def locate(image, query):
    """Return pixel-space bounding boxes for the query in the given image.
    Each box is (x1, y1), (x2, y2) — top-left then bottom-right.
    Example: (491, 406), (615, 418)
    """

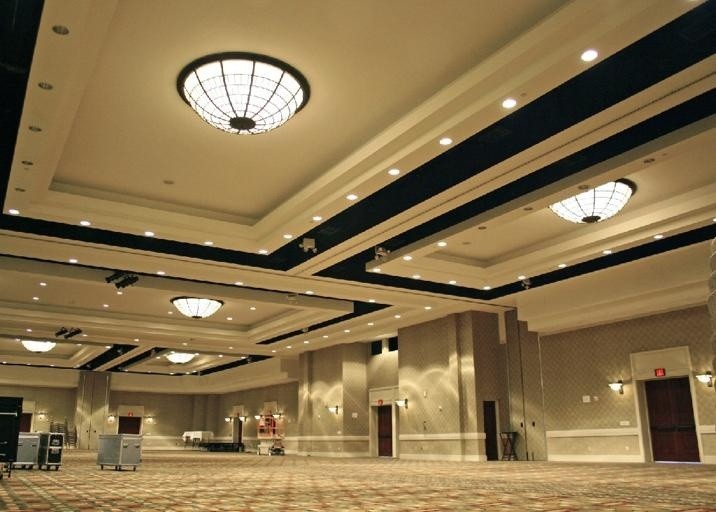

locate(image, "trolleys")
(1, 412), (64, 478)
(96, 432), (143, 471)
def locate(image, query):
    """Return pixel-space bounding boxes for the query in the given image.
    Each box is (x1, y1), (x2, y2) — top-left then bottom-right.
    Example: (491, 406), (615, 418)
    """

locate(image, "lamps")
(607, 379), (625, 395)
(175, 50), (311, 136)
(545, 177), (639, 225)
(696, 371), (713, 387)
(169, 296), (225, 320)
